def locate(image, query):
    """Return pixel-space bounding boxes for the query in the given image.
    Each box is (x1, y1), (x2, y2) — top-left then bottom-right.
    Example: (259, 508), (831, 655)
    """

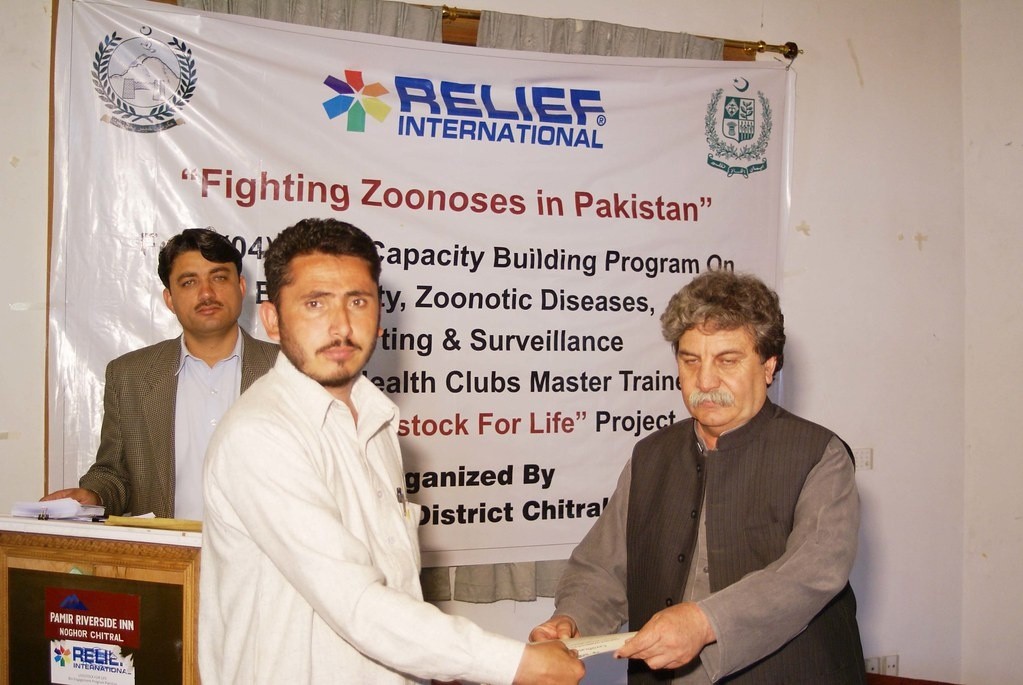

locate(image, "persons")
(39, 229), (281, 522)
(196, 218), (586, 685)
(528, 272), (866, 685)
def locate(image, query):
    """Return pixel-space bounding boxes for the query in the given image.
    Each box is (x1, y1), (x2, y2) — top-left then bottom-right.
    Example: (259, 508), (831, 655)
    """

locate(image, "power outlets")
(864, 654), (898, 677)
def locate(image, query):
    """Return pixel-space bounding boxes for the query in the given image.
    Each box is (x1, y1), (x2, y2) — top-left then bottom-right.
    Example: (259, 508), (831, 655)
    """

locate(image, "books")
(533, 631), (638, 659)
